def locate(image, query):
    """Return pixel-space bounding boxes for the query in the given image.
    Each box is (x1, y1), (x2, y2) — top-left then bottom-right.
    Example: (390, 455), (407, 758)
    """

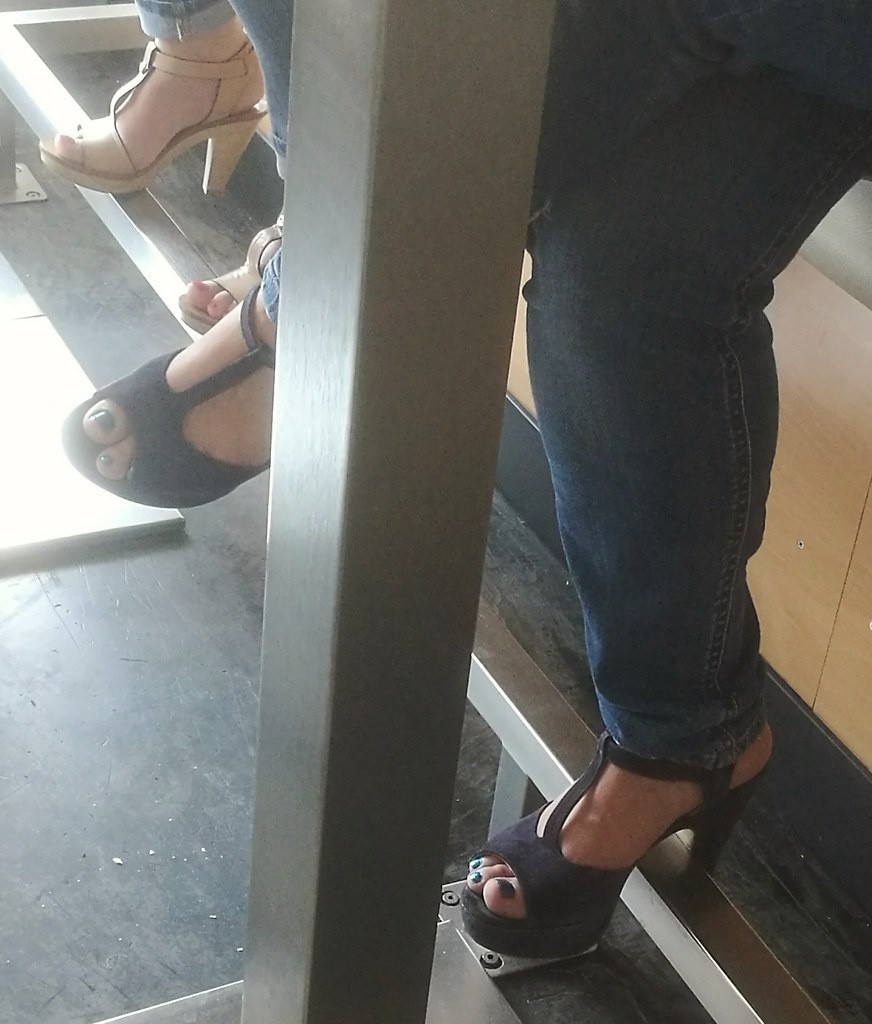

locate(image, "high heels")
(461, 716), (774, 957)
(63, 287), (275, 510)
(178, 216), (284, 336)
(39, 35), (271, 198)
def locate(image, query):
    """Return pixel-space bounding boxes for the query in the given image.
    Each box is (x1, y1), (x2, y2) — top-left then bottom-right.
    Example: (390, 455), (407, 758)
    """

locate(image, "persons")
(61, 0), (872, 961)
(38, 0), (293, 337)
